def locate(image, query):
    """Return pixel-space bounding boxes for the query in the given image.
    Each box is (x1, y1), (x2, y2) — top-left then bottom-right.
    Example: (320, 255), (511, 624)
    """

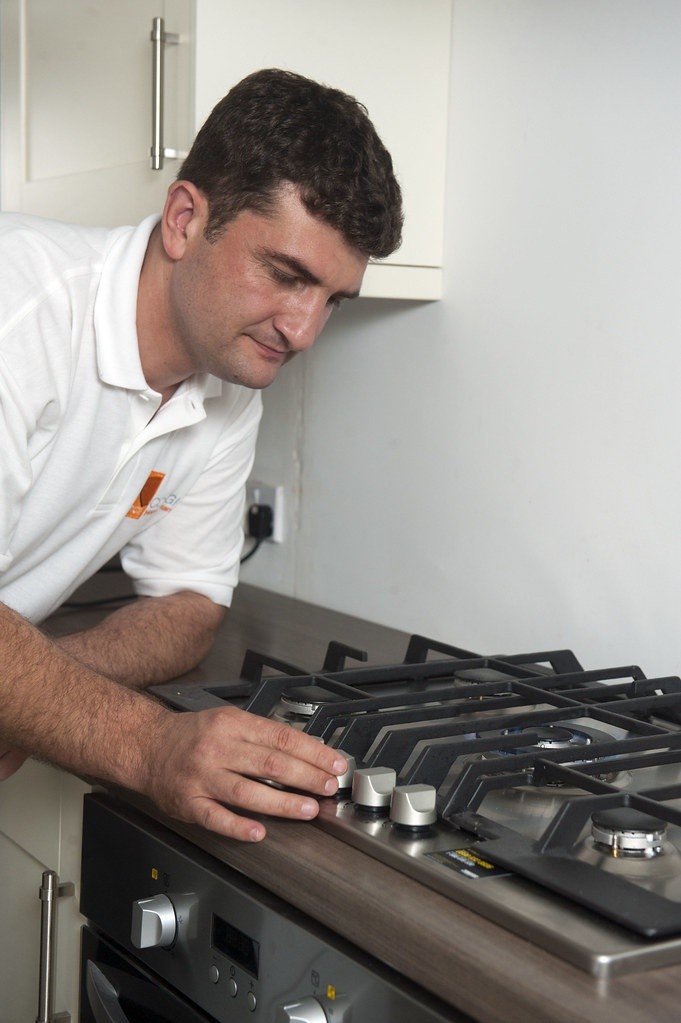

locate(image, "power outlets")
(239, 477), (287, 545)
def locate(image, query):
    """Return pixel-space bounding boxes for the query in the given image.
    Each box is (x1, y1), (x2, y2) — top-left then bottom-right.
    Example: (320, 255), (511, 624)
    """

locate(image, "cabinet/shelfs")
(1, 559), (451, 1023)
(0, 1), (446, 303)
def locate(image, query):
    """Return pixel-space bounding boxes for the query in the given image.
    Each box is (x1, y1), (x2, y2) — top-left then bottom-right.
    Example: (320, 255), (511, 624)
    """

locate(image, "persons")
(0, 67), (405, 844)
